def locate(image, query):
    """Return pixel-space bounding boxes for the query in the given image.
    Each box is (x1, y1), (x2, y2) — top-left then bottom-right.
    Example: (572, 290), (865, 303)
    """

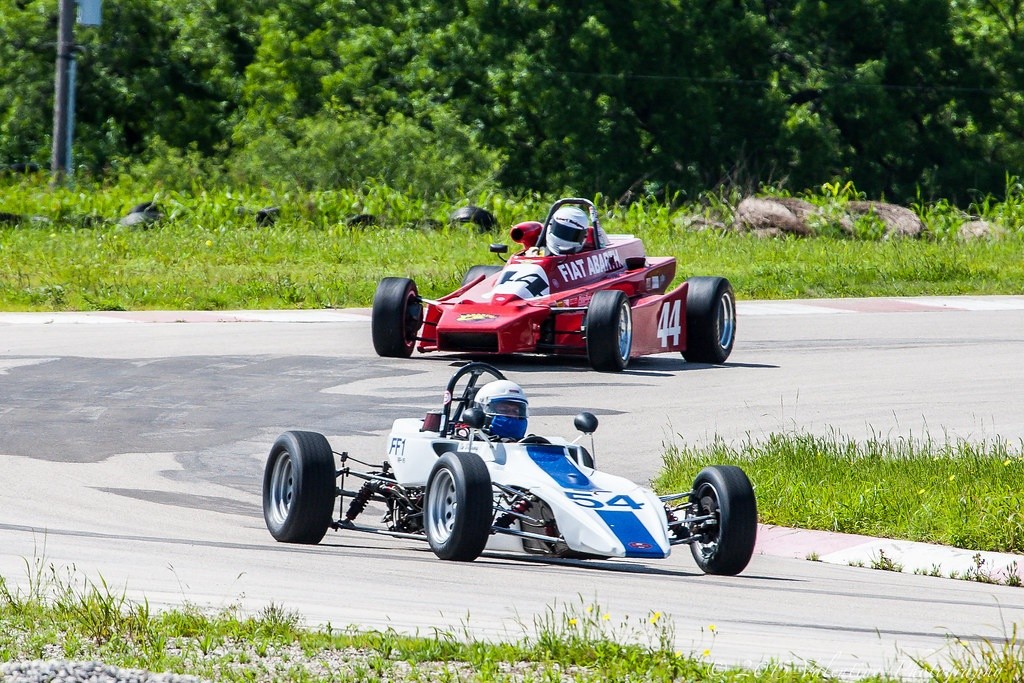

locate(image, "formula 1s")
(262, 363), (758, 577)
(372, 197), (736, 374)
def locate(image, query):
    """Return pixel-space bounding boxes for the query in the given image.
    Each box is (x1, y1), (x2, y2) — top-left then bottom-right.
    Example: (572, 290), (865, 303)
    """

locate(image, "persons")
(525, 207), (589, 257)
(454, 380), (528, 441)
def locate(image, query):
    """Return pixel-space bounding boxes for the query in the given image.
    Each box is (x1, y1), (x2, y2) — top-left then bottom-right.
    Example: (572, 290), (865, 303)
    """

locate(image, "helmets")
(546, 206), (589, 256)
(473, 380), (529, 440)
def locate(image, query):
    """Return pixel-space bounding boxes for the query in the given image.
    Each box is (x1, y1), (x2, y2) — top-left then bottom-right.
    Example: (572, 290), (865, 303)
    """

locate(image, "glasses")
(494, 408), (520, 414)
